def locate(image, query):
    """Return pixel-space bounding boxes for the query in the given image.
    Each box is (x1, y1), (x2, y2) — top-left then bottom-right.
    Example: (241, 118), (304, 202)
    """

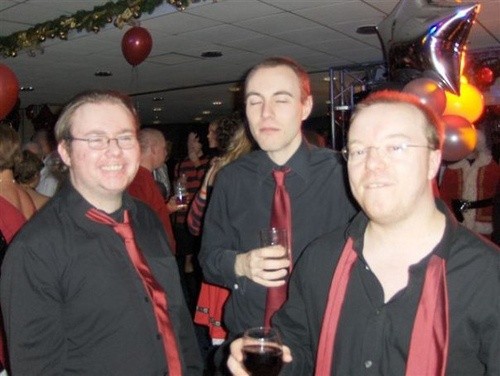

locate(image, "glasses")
(67, 134), (136, 150)
(341, 142), (432, 162)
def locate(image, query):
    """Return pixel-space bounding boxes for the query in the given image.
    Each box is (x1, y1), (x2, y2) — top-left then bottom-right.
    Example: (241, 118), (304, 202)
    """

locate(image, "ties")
(264, 168), (293, 330)
(86, 208), (181, 376)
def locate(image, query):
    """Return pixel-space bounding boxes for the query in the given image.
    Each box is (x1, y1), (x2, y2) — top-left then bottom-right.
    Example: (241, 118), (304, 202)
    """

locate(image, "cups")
(242, 327), (284, 376)
(260, 226), (288, 271)
(176, 193), (189, 210)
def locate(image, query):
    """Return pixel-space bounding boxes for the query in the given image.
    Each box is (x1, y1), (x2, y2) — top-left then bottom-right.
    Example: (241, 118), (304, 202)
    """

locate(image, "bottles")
(176, 174), (187, 193)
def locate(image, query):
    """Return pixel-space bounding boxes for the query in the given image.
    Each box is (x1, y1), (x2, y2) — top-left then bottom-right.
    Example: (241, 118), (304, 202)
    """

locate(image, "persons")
(0, 89), (203, 376)
(0, 110), (259, 376)
(213, 91), (499, 376)
(198, 57), (362, 341)
(440, 125), (499, 241)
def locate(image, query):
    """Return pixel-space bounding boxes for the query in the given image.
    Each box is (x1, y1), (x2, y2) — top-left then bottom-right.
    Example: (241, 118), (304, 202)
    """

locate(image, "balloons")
(375, 1), (497, 162)
